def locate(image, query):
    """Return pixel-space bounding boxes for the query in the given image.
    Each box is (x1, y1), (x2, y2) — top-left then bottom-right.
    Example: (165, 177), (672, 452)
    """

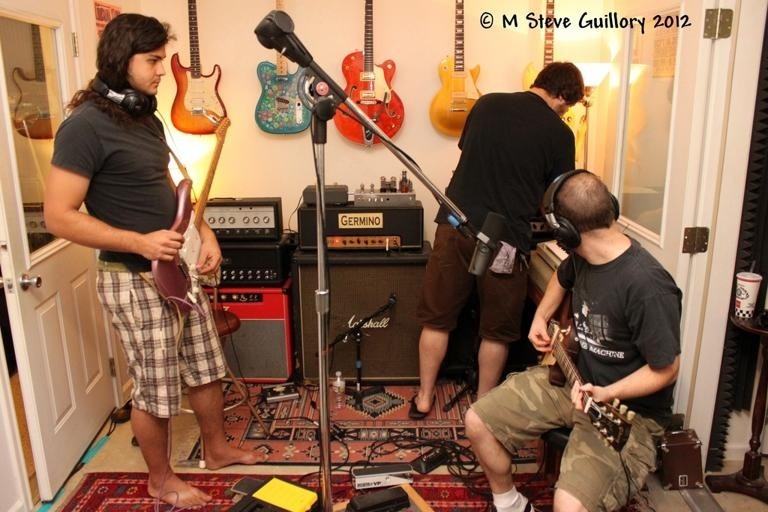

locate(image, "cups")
(736, 273), (763, 320)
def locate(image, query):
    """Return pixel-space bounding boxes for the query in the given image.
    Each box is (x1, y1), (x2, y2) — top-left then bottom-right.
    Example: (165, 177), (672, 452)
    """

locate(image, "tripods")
(315, 304), (385, 411)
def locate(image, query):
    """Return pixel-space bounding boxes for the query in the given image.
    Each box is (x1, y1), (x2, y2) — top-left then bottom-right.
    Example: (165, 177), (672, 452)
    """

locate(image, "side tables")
(705, 301), (768, 504)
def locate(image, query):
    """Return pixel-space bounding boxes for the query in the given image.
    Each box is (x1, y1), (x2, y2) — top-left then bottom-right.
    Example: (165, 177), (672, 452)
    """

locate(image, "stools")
(540, 427), (575, 479)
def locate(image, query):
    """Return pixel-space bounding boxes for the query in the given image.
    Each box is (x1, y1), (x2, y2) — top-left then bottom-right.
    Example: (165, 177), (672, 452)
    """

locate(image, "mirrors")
(1, 8), (78, 271)
(610, 4), (685, 250)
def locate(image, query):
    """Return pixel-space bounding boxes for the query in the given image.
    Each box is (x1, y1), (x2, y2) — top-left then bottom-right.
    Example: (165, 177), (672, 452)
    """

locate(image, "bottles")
(333, 371), (347, 410)
(380, 170), (410, 193)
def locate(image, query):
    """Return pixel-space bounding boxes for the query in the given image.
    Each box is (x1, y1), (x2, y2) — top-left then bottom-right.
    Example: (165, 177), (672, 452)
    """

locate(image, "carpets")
(173, 381), (545, 464)
(47, 471), (656, 512)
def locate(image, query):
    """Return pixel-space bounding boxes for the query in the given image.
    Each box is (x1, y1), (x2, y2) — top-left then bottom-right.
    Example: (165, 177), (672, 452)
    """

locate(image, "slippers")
(408, 393), (436, 420)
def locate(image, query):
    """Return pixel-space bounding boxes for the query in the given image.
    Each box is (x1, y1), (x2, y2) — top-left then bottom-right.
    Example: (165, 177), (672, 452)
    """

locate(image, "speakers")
(293, 240), (433, 386)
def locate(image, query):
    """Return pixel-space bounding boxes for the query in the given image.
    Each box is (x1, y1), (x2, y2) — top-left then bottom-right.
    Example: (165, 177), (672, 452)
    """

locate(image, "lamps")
(629, 63), (647, 84)
(575, 61), (611, 170)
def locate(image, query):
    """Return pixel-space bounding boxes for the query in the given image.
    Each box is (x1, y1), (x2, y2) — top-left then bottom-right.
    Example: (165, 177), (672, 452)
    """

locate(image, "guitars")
(254, 1), (314, 134)
(521, 0), (577, 127)
(430, 0), (483, 138)
(335, 0), (404, 146)
(169, 1), (227, 136)
(536, 316), (636, 452)
(12, 23), (54, 140)
(148, 117), (232, 311)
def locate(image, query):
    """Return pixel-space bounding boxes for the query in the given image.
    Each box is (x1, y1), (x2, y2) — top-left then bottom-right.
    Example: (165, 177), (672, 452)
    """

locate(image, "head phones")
(91, 70), (157, 118)
(543, 168), (619, 250)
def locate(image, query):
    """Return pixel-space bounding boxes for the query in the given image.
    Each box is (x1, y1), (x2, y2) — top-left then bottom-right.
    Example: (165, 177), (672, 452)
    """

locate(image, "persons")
(464, 167), (685, 512)
(41, 13), (265, 511)
(405, 60), (588, 425)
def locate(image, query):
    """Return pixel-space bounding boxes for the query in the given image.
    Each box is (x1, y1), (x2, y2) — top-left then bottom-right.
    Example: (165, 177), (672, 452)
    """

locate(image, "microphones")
(467, 211), (509, 278)
(388, 296), (396, 310)
